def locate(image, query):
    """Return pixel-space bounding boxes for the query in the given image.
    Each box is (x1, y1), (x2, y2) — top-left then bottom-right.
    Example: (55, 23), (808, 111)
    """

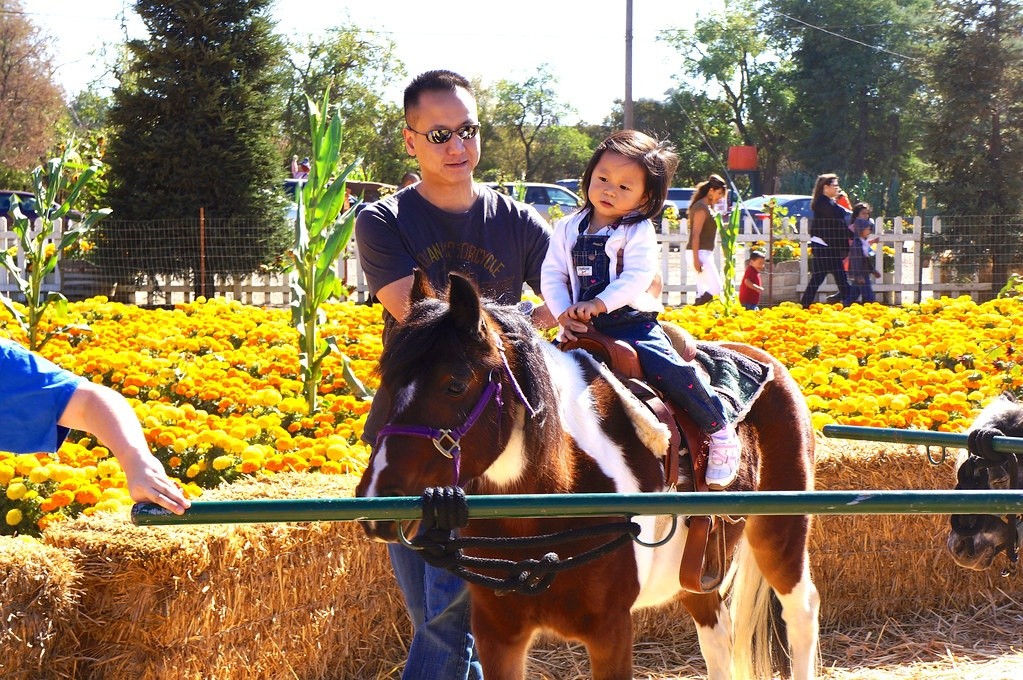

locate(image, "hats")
(299, 157), (310, 169)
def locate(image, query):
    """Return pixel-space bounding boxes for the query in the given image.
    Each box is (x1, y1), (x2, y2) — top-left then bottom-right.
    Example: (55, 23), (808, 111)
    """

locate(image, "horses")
(948, 395), (1023, 573)
(356, 267), (820, 680)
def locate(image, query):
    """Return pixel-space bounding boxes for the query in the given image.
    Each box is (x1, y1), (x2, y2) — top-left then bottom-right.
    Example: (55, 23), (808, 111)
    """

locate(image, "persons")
(540, 130), (743, 490)
(799, 172), (857, 310)
(738, 251), (767, 311)
(847, 217), (880, 307)
(355, 69), (555, 680)
(291, 155), (311, 180)
(824, 204), (880, 302)
(0, 336), (191, 515)
(401, 172), (419, 188)
(686, 174), (727, 301)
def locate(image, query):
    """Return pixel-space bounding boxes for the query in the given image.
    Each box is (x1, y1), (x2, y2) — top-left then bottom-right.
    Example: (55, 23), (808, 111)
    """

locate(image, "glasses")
(406, 124), (482, 144)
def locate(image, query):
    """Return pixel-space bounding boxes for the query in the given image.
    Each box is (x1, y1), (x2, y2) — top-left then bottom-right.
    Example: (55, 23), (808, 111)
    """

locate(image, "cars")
(730, 190), (875, 248)
(255, 176), (357, 252)
(0, 190), (86, 233)
(483, 174), (700, 241)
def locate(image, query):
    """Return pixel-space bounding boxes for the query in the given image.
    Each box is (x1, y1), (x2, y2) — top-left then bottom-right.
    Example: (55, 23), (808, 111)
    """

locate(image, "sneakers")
(705, 431), (743, 492)
